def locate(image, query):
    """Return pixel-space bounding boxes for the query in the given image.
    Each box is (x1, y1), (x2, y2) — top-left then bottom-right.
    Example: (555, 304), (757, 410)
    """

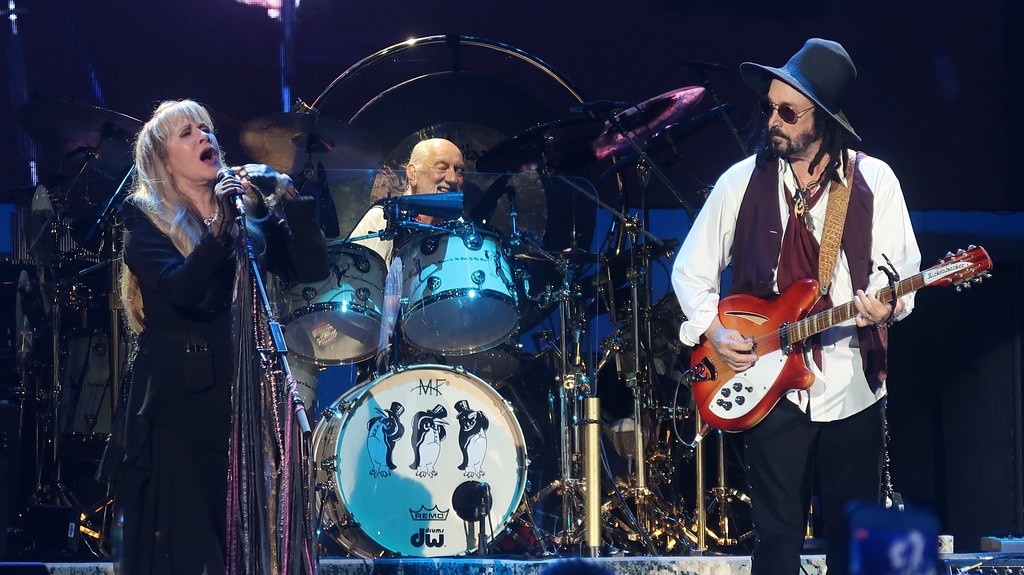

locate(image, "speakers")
(0, 399), (21, 561)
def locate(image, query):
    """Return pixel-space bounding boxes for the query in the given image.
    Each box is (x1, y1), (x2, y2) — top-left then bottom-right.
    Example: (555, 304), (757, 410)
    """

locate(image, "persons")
(94, 99), (330, 575)
(344, 138), (465, 271)
(670, 38), (922, 575)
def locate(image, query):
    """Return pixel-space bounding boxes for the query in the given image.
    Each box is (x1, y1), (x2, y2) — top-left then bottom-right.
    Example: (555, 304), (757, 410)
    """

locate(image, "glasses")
(761, 92), (818, 124)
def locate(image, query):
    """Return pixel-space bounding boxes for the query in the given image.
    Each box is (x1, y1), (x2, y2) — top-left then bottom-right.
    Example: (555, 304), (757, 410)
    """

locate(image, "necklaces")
(791, 158), (831, 219)
(204, 214), (214, 225)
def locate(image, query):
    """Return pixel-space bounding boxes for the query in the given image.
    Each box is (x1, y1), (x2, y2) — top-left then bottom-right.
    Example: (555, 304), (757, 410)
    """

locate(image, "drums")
(394, 222), (522, 354)
(306, 361), (531, 556)
(277, 244), (389, 367)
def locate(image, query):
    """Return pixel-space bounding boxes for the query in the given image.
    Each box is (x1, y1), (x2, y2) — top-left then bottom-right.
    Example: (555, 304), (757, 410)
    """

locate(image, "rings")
(866, 314), (870, 319)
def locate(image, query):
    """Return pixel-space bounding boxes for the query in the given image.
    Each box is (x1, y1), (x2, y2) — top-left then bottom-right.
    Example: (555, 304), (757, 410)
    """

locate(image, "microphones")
(469, 170), (513, 225)
(461, 481), (490, 509)
(316, 163), (340, 238)
(570, 100), (636, 112)
(680, 59), (728, 73)
(217, 167), (246, 216)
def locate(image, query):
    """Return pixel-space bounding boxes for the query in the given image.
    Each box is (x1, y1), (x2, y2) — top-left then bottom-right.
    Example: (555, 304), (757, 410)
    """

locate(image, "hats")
(740, 36), (862, 141)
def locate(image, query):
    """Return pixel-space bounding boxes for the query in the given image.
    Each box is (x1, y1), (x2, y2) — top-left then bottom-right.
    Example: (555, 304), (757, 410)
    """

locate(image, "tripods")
(10, 122), (113, 560)
(486, 158), (755, 561)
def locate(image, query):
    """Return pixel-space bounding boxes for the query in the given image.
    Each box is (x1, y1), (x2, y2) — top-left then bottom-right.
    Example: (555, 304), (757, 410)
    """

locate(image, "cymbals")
(476, 117), (605, 174)
(588, 88), (707, 159)
(14, 99), (146, 163)
(238, 108), (375, 181)
(579, 244), (677, 295)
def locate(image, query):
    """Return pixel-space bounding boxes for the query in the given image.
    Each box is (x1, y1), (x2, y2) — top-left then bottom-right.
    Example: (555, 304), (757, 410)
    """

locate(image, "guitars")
(689, 245), (992, 429)
